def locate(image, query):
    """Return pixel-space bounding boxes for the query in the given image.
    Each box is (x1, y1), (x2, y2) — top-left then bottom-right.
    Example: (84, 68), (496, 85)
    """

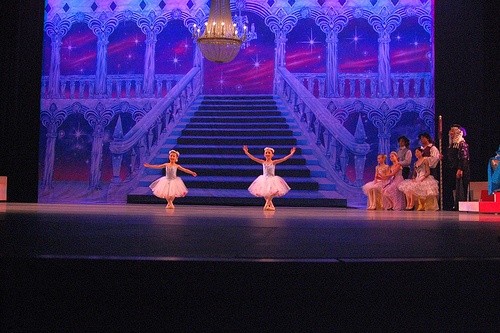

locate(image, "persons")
(440, 124), (471, 210)
(397, 135), (412, 179)
(378, 151), (406, 211)
(361, 154), (389, 210)
(144, 149), (198, 209)
(398, 147), (439, 211)
(243, 145), (296, 211)
(418, 132), (439, 181)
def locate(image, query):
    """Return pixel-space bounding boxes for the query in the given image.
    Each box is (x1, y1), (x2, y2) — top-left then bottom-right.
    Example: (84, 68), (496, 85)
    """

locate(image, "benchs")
(458, 190), (500, 215)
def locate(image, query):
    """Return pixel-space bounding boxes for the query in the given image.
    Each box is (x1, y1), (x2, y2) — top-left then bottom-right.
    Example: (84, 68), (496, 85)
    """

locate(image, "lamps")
(193, 0), (247, 64)
